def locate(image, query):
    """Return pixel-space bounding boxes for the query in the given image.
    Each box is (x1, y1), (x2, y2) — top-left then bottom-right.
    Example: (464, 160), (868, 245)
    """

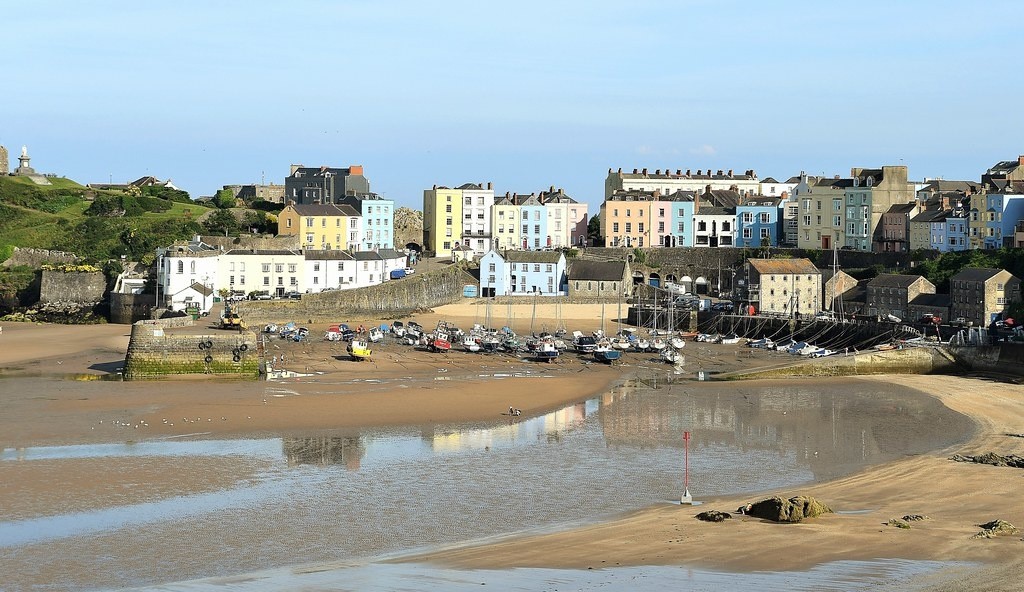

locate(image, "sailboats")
(659, 278), (681, 363)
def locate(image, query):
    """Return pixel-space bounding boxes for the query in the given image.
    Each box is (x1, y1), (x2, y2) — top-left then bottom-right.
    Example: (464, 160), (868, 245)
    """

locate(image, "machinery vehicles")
(219, 294), (249, 331)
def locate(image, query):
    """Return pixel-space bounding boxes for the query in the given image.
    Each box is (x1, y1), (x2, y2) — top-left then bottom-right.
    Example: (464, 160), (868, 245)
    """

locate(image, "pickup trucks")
(918, 313), (942, 325)
(949, 317), (974, 328)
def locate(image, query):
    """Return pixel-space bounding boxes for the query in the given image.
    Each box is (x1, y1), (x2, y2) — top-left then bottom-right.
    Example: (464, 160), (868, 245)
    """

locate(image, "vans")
(390, 269), (406, 279)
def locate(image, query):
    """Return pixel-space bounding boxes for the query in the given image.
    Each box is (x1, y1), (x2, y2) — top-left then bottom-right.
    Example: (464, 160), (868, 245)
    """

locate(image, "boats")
(264, 321), (313, 343)
(532, 327), (560, 361)
(346, 334), (373, 359)
(325, 277), (837, 357)
(591, 344), (622, 361)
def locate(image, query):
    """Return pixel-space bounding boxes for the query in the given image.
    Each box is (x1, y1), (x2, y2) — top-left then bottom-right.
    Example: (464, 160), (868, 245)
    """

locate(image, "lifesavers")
(233, 355), (241, 362)
(233, 349), (240, 355)
(206, 341), (213, 348)
(198, 343), (206, 350)
(205, 355), (213, 364)
(240, 344), (248, 352)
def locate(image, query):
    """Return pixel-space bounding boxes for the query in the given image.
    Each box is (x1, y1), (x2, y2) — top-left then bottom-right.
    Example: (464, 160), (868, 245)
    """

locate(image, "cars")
(253, 292), (275, 301)
(403, 267), (416, 275)
(282, 290), (305, 300)
(180, 307), (210, 317)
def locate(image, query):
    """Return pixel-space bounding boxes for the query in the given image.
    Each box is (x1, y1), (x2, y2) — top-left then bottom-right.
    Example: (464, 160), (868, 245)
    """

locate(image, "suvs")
(226, 292), (248, 301)
